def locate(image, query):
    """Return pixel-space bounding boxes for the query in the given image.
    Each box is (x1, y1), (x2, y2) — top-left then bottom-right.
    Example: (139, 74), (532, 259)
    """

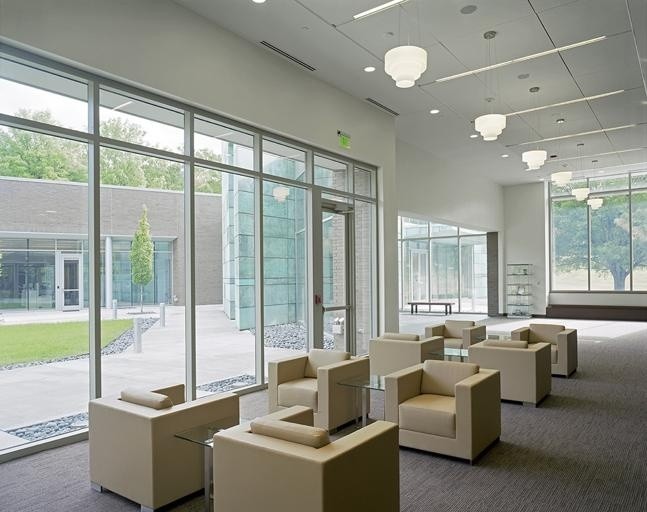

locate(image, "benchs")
(407, 300), (455, 316)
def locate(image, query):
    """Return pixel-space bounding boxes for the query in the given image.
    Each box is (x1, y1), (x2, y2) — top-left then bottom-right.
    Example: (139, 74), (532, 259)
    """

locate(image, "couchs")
(367, 332), (444, 390)
(266, 347), (370, 437)
(381, 358), (502, 467)
(211, 405), (402, 511)
(466, 337), (552, 409)
(423, 319), (486, 351)
(509, 323), (578, 379)
(85, 382), (240, 512)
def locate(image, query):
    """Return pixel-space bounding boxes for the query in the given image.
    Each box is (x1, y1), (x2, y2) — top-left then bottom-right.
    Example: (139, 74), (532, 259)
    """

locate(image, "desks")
(171, 413), (255, 512)
(425, 347), (468, 363)
(334, 371), (390, 433)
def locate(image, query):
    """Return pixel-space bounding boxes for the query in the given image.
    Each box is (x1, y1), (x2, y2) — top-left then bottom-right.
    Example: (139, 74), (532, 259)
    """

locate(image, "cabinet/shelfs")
(504, 262), (534, 318)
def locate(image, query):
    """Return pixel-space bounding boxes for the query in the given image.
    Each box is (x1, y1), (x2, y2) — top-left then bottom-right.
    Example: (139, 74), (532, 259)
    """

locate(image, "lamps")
(471, 135), (479, 140)
(383, 1), (428, 89)
(520, 125), (634, 146)
(573, 144), (591, 202)
(551, 119), (573, 188)
(436, 37), (604, 82)
(474, 31), (507, 141)
(353, 1), (404, 21)
(430, 110), (439, 115)
(506, 90), (624, 116)
(502, 155), (508, 159)
(522, 87), (547, 171)
(588, 159), (604, 211)
